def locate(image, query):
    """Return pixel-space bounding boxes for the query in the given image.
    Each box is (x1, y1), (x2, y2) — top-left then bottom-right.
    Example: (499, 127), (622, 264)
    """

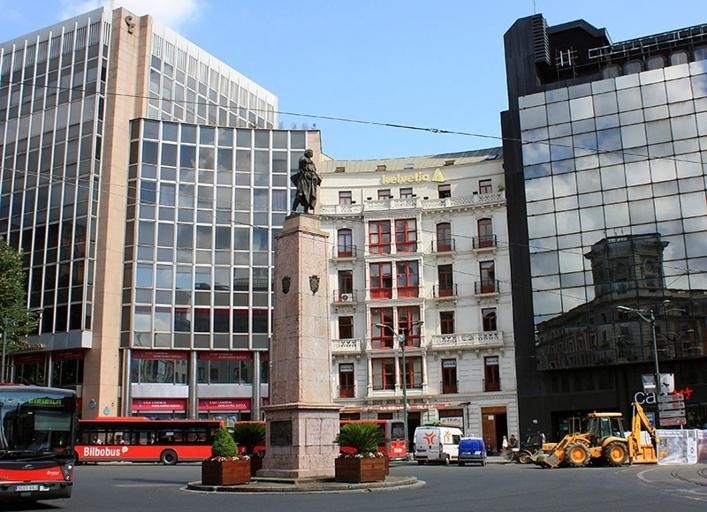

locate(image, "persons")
(292, 149), (322, 213)
(91, 435), (102, 445)
(24, 431), (49, 451)
(502, 428), (546, 450)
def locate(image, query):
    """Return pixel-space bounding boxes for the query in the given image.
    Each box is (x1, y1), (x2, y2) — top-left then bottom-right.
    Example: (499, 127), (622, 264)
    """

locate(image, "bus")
(0, 383), (77, 506)
(74, 416), (266, 465)
(339, 419), (409, 463)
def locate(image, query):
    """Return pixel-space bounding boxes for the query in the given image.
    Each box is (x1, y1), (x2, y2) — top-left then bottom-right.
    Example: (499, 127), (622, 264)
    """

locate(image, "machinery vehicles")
(530, 401), (668, 469)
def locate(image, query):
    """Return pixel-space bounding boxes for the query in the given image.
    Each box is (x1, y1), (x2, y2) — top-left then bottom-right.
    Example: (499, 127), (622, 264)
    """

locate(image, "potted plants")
(201, 423), (267, 484)
(333, 424), (388, 483)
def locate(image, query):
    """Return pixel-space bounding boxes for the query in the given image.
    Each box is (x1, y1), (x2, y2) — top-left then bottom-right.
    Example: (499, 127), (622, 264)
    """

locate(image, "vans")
(413, 426), (465, 466)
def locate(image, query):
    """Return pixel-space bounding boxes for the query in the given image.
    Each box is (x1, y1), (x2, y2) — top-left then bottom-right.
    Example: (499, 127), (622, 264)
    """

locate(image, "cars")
(458, 436), (487, 467)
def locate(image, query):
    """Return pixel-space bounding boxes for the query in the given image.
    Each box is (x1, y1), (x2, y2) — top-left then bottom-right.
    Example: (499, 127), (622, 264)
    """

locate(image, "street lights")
(373, 319), (424, 442)
(615, 300), (686, 401)
